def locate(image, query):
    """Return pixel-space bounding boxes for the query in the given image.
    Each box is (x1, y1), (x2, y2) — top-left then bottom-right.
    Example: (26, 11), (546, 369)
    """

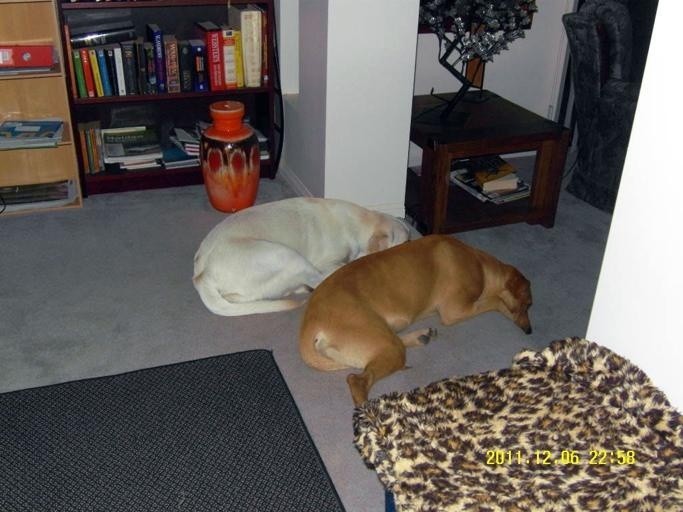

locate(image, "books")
(0, 118), (64, 148)
(1, 179), (74, 211)
(0, 45), (54, 75)
(63, 4), (269, 98)
(78, 118), (270, 173)
(450, 155), (531, 206)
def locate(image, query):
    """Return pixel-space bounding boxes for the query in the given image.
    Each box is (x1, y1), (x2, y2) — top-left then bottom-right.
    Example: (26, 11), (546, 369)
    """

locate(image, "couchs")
(560, 0), (640, 218)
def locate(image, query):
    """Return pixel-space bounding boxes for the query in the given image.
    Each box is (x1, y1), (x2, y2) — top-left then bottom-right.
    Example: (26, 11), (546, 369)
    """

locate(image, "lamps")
(409, 1), (540, 147)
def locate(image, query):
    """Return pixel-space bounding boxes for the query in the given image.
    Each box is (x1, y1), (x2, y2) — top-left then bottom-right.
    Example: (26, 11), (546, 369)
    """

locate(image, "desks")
(409, 89), (572, 234)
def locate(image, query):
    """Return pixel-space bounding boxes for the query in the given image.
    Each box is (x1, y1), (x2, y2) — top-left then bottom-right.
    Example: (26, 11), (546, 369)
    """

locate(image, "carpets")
(0, 349), (348, 512)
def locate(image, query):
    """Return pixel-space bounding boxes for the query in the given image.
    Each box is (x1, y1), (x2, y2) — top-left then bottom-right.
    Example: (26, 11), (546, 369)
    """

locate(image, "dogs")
(299, 234), (535, 408)
(190, 195), (428, 317)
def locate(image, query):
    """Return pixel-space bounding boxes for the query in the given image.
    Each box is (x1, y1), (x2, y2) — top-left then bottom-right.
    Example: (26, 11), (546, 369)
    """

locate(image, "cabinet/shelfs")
(0, 3), (84, 217)
(56, 1), (284, 188)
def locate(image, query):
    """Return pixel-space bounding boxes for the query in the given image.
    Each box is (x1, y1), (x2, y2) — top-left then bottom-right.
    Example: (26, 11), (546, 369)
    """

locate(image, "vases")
(197, 96), (260, 213)
